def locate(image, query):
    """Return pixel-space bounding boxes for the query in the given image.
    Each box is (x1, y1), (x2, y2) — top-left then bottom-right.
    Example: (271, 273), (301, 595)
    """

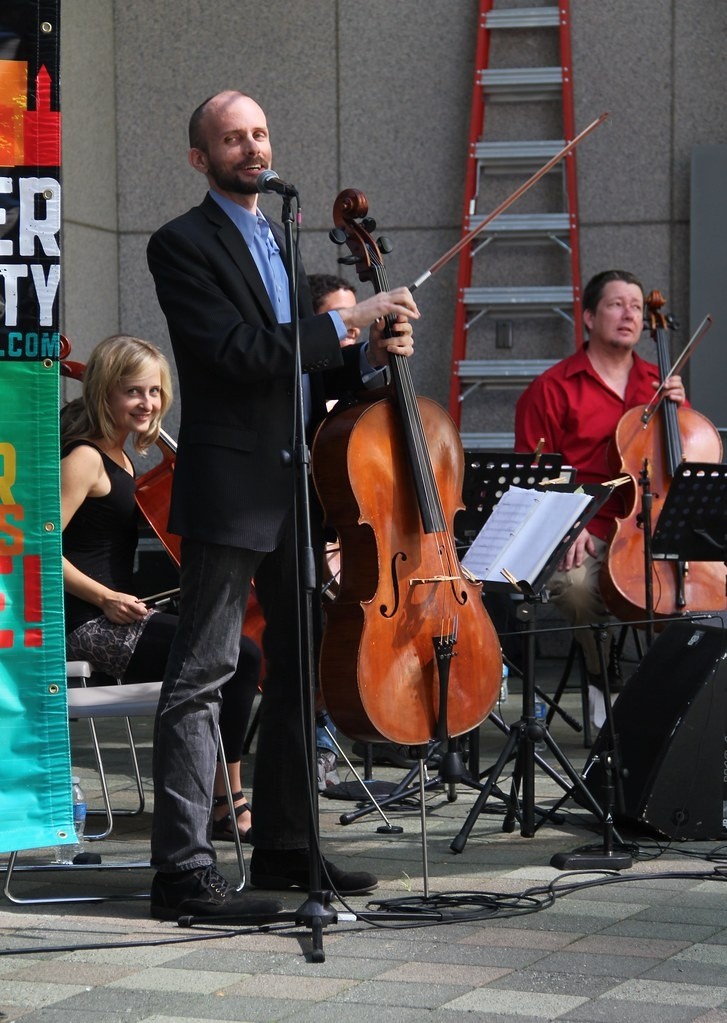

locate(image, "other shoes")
(315, 748), (341, 791)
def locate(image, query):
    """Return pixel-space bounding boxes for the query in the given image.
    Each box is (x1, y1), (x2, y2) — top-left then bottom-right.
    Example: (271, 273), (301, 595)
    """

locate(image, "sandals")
(352, 739), (443, 770)
(211, 791), (253, 843)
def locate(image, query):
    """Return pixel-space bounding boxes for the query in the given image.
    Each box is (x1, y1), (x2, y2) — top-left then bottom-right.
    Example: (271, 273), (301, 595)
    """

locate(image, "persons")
(512, 268), (692, 731)
(300, 271), (444, 792)
(148, 87), (423, 917)
(59, 334), (254, 842)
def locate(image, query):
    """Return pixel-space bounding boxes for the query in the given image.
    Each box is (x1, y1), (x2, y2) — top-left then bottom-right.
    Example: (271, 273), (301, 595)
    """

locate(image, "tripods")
(339, 586), (624, 854)
(177, 197), (443, 963)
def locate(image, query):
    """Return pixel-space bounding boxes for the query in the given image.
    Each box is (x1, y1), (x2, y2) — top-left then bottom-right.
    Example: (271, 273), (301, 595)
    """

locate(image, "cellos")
(58, 335), (398, 834)
(601, 292), (727, 621)
(311, 190), (505, 901)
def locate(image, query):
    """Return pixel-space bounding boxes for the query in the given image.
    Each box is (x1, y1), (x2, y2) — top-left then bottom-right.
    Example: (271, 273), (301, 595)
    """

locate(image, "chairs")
(2, 657), (249, 907)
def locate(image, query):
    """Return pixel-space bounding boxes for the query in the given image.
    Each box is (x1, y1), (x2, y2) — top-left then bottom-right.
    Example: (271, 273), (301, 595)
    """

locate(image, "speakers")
(578, 621), (727, 842)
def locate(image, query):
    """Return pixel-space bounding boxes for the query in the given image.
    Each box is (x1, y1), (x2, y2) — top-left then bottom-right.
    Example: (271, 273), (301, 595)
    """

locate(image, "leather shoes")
(151, 866), (282, 923)
(250, 846), (381, 895)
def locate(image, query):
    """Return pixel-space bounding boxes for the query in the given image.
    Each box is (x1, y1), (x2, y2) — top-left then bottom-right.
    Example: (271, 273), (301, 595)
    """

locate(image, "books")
(459, 484), (594, 589)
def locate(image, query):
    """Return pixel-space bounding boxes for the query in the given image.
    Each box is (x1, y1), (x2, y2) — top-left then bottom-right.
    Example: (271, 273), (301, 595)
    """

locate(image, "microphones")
(256, 167), (297, 200)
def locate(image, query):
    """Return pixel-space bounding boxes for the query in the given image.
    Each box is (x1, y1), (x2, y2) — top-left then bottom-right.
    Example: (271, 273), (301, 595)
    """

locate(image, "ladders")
(435, 1), (588, 458)
